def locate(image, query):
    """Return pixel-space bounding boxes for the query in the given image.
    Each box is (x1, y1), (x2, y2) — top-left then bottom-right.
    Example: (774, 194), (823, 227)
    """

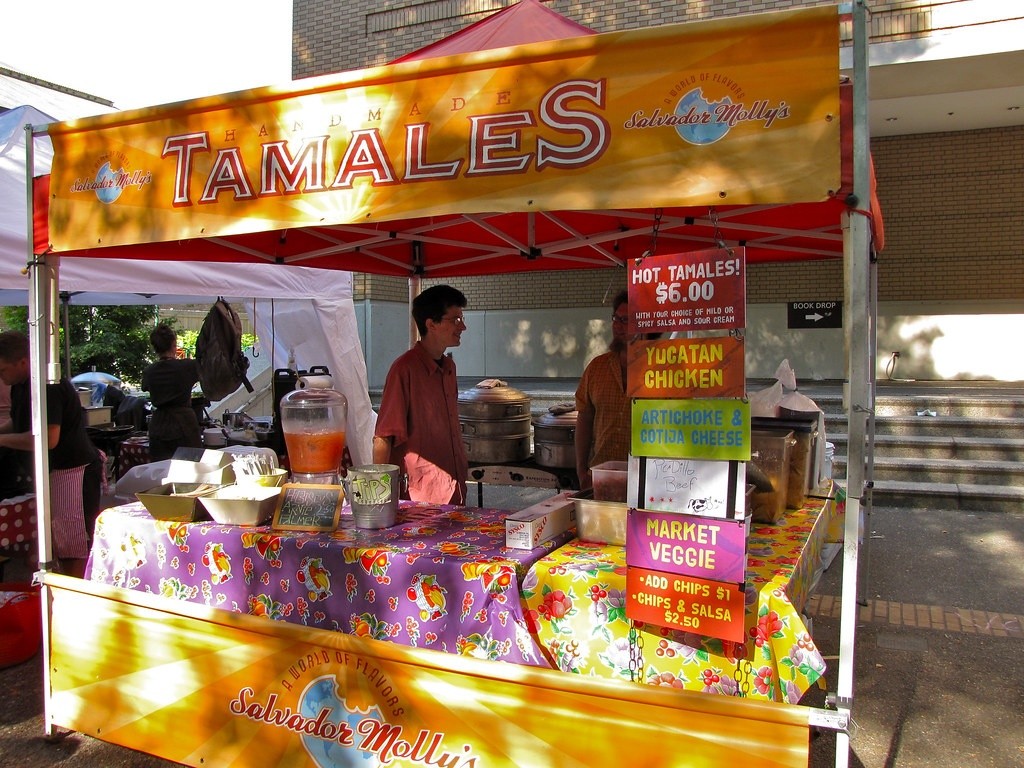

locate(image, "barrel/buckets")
(234, 468), (289, 486)
(346, 463), (402, 529)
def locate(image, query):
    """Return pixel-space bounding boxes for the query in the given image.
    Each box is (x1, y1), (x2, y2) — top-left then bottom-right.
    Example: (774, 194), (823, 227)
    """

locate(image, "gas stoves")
(455, 453), (581, 491)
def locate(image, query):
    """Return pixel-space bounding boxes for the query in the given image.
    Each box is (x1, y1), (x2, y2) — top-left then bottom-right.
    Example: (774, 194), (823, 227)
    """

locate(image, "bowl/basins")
(256, 430), (274, 440)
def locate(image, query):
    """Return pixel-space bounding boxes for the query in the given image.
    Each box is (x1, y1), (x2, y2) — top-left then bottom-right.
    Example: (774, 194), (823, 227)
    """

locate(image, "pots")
(223, 409), (244, 427)
(531, 410), (579, 469)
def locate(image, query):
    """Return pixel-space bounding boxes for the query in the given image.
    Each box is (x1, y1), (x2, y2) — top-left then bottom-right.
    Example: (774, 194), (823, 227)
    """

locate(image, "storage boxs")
(590, 461), (628, 503)
(567, 488), (629, 547)
(503, 492), (575, 550)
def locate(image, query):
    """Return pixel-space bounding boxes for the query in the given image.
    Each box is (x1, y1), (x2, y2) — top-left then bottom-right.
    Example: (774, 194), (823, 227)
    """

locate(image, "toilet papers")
(295, 375), (334, 391)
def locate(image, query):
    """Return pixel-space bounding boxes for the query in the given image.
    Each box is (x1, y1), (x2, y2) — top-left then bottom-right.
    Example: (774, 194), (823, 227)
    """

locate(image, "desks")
(83, 500), (573, 670)
(519, 479), (846, 704)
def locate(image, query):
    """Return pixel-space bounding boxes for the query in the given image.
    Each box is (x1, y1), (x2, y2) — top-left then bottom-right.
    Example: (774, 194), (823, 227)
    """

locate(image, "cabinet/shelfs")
(746, 429), (797, 523)
(751, 416), (819, 509)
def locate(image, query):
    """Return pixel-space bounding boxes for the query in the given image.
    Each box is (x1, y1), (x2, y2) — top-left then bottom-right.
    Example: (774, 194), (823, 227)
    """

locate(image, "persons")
(140, 323), (203, 465)
(0, 329), (102, 579)
(575, 291), (663, 493)
(373, 282), (469, 506)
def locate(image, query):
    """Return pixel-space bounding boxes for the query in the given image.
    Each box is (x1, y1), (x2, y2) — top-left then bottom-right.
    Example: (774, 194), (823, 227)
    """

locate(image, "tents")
(0, 8), (885, 768)
